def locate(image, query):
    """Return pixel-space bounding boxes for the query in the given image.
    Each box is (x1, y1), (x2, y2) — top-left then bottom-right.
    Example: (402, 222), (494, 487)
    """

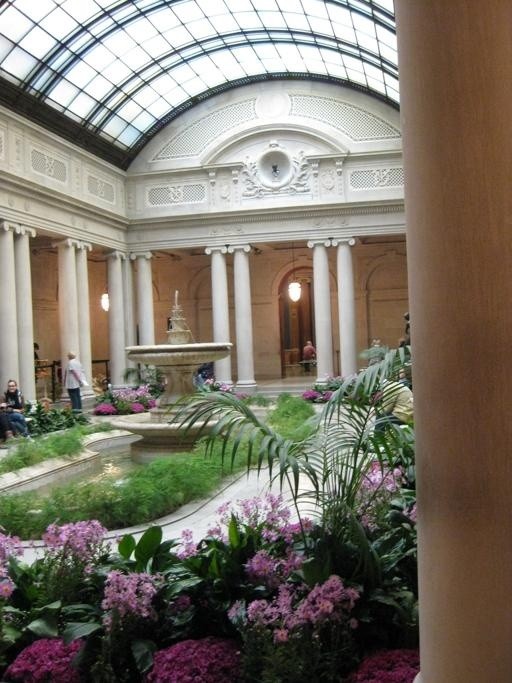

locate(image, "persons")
(65, 350), (89, 414)
(1, 378), (35, 442)
(302, 340), (316, 360)
(34, 341), (42, 383)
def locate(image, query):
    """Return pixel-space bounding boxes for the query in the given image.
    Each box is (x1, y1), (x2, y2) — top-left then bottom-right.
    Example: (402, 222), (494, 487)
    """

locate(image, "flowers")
(0, 457), (420, 682)
(92, 378), (382, 415)
